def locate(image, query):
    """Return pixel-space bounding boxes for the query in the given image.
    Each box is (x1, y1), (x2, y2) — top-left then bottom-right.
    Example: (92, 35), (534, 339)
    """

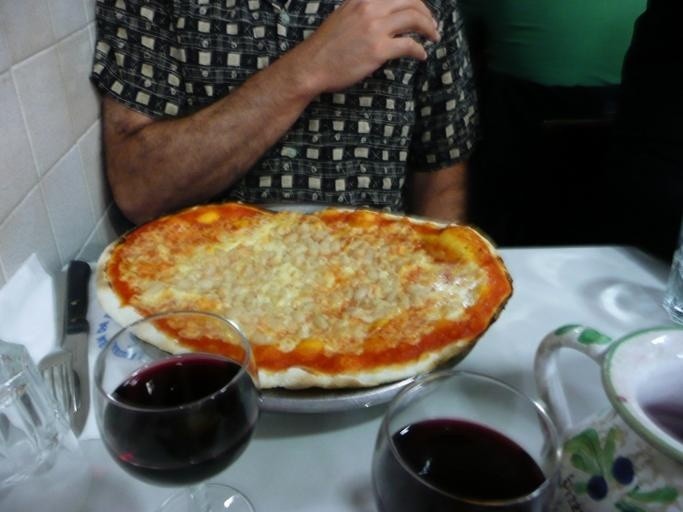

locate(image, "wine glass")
(91, 306), (264, 511)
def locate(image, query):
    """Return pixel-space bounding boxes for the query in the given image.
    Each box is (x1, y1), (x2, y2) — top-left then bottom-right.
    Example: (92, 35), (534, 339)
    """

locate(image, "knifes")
(57, 259), (93, 443)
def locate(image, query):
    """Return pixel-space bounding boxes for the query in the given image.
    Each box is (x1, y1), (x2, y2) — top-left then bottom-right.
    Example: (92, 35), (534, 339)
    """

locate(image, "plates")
(127, 196), (493, 416)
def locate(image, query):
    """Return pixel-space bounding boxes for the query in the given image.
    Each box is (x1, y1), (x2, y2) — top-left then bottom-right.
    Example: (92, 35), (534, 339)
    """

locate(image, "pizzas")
(93, 202), (514, 389)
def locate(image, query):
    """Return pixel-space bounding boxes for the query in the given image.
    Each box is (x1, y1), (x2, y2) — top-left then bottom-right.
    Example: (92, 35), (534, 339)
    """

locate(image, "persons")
(92, 0), (476, 230)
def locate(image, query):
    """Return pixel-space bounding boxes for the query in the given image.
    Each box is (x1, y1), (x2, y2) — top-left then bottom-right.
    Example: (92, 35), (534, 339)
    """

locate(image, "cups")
(530, 323), (682, 511)
(367, 368), (567, 512)
